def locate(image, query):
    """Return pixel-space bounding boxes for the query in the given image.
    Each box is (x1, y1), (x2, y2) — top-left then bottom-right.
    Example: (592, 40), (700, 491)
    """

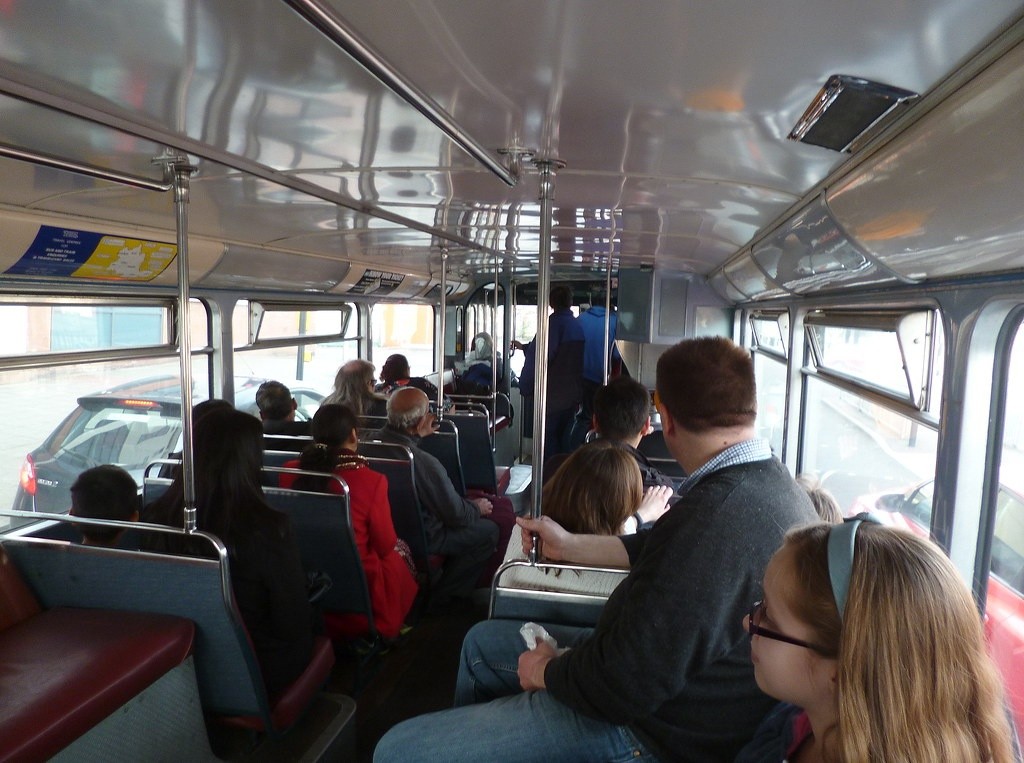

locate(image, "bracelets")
(634, 512), (643, 528)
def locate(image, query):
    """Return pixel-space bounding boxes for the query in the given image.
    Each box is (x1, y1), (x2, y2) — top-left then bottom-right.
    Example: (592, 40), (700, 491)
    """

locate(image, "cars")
(847, 468), (1024, 763)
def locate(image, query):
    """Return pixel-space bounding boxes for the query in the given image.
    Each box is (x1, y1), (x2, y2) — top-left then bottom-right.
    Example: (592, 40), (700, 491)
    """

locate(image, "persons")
(570, 293), (621, 445)
(509, 286), (585, 456)
(505, 438), (673, 564)
(593, 376), (674, 490)
(192, 399), (230, 420)
(372, 335), (821, 763)
(256, 380), (313, 450)
(374, 354), (456, 414)
(70, 466), (139, 547)
(451, 364), (509, 418)
(277, 404), (425, 637)
(317, 359), (388, 417)
(742, 520), (1016, 763)
(144, 407), (324, 695)
(800, 478), (843, 524)
(368, 388), (501, 586)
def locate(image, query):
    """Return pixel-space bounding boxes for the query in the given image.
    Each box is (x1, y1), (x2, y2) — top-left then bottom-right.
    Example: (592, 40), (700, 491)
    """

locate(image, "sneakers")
(428, 594), (490, 618)
(373, 639), (393, 655)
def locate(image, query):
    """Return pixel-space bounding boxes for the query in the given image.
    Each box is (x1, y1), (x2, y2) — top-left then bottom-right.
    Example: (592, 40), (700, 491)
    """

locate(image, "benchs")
(0, 367), (691, 763)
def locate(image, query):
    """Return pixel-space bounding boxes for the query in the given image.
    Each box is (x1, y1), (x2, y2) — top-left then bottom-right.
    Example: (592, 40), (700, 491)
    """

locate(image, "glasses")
(749, 600), (840, 659)
(371, 380), (377, 383)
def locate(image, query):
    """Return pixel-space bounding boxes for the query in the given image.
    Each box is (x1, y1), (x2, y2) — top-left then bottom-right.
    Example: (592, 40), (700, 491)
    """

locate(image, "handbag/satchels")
(394, 537), (419, 579)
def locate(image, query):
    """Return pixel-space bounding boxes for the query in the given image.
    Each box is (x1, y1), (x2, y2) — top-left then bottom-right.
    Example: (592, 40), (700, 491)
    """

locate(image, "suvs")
(9, 373), (326, 532)
